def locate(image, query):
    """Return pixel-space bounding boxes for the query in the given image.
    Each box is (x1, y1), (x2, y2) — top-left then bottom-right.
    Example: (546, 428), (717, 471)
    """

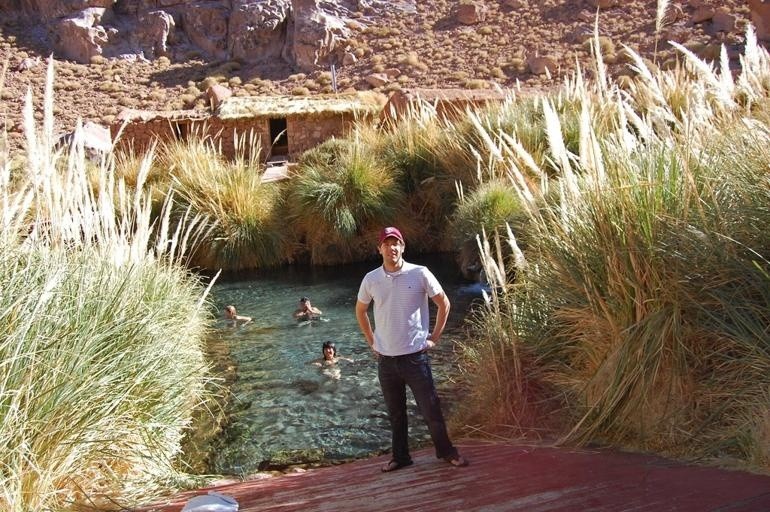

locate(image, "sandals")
(448, 454), (470, 468)
(381, 458), (413, 473)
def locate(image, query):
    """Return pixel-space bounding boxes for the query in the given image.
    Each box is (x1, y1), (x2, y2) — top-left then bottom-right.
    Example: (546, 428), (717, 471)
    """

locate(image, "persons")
(356, 226), (469, 472)
(293, 296), (323, 320)
(215, 305), (254, 326)
(304, 339), (356, 375)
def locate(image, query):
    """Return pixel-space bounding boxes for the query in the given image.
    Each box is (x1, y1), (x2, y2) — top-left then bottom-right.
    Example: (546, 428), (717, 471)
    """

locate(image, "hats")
(379, 226), (404, 243)
